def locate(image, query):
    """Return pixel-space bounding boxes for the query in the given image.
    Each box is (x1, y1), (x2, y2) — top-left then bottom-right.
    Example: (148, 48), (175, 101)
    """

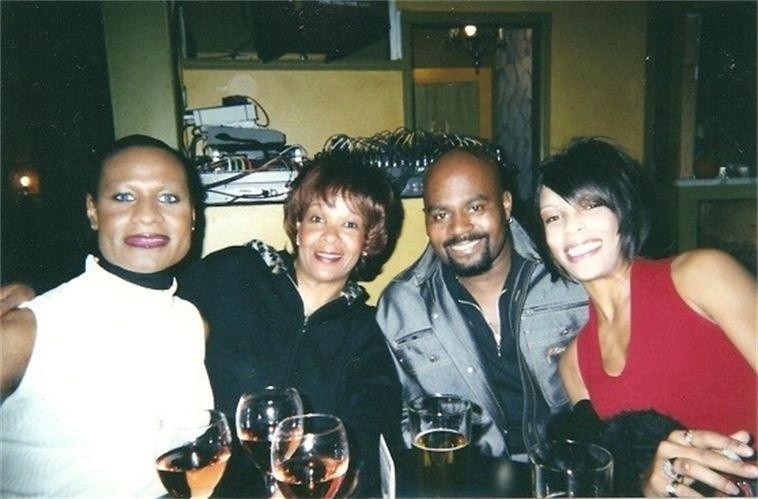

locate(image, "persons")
(0, 147), (403, 499)
(0, 134), (214, 499)
(529, 135), (758, 499)
(375, 145), (590, 464)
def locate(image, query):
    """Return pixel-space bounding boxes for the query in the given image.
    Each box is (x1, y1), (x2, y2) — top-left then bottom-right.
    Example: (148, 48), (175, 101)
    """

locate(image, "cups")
(271, 419), (348, 499)
(409, 392), (475, 497)
(530, 439), (616, 499)
(155, 410), (231, 499)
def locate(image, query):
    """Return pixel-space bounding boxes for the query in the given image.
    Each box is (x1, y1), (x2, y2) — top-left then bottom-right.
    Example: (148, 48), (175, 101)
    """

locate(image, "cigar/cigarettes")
(721, 446), (743, 462)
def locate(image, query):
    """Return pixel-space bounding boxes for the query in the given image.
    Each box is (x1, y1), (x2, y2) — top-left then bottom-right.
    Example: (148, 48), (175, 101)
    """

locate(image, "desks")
(204, 442), (542, 499)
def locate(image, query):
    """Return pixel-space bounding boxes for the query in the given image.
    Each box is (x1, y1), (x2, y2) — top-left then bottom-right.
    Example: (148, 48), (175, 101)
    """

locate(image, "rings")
(666, 479), (679, 497)
(661, 457), (682, 481)
(683, 428), (693, 447)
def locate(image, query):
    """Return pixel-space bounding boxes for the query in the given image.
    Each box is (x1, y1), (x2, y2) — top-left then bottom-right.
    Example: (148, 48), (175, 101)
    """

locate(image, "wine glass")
(236, 388), (304, 494)
